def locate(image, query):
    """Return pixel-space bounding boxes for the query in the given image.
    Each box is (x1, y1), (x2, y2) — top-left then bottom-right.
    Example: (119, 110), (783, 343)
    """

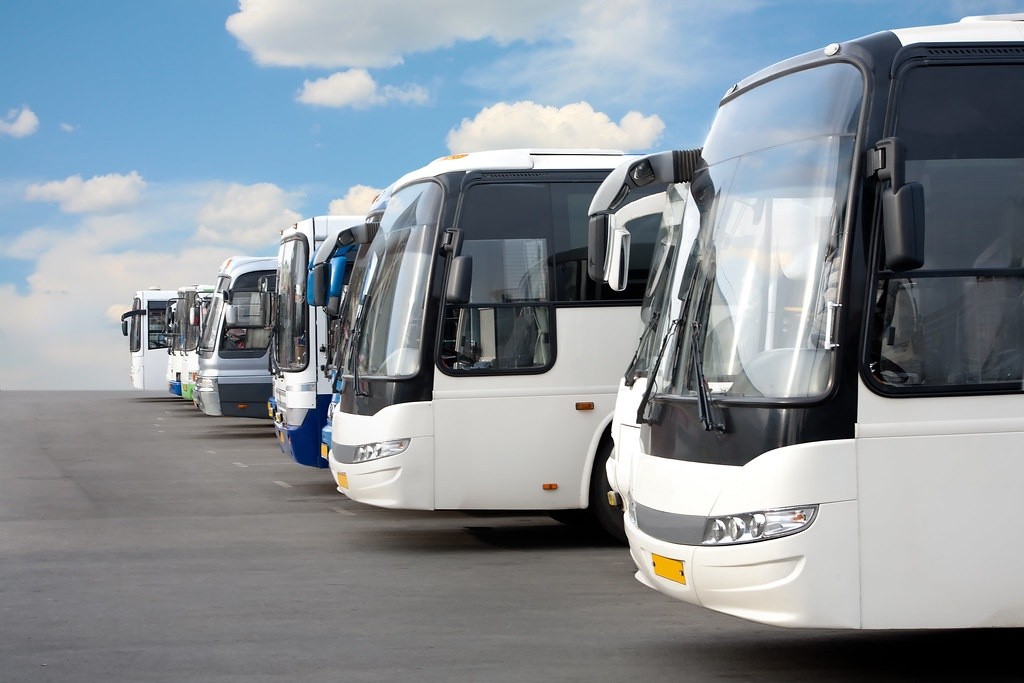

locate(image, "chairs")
(558, 260), (581, 302)
(500, 313), (538, 368)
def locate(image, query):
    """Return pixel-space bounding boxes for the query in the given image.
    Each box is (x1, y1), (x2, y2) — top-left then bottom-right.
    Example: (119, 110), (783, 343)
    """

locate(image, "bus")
(165, 285), (264, 402)
(120, 286), (177, 390)
(307, 148), (823, 544)
(189, 256), (277, 419)
(584, 16), (1024, 627)
(257, 213), (547, 470)
